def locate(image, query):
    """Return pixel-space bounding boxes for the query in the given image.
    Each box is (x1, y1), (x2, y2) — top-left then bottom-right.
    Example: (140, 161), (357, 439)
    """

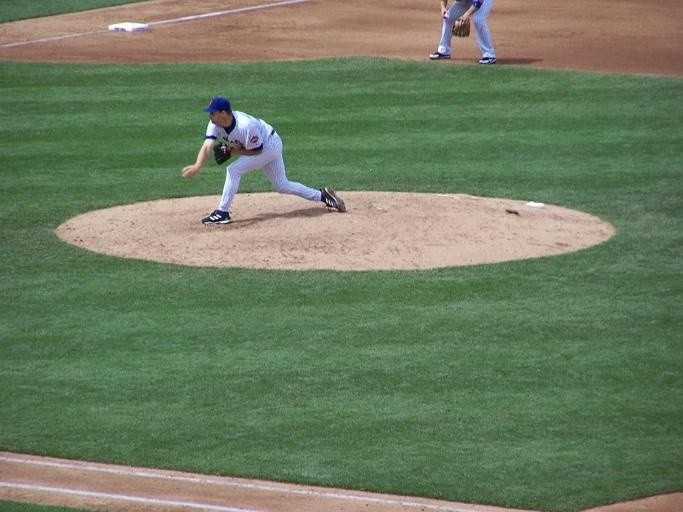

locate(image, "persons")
(182, 96), (346, 226)
(428, 0), (497, 64)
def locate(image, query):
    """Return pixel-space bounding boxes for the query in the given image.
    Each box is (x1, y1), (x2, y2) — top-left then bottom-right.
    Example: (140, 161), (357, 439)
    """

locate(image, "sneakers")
(202, 209), (231, 224)
(322, 187), (346, 212)
(478, 58), (496, 64)
(429, 51), (451, 60)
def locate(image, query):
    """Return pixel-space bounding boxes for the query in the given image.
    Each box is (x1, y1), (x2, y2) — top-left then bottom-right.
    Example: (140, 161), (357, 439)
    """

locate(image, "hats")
(203, 98), (230, 113)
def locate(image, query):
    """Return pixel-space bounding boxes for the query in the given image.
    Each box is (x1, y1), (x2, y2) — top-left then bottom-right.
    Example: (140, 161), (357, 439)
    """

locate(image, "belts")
(271, 130), (275, 136)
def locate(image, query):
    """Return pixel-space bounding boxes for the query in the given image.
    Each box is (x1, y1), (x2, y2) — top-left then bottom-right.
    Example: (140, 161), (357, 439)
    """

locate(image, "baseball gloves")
(213, 143), (231, 164)
(452, 16), (470, 37)
